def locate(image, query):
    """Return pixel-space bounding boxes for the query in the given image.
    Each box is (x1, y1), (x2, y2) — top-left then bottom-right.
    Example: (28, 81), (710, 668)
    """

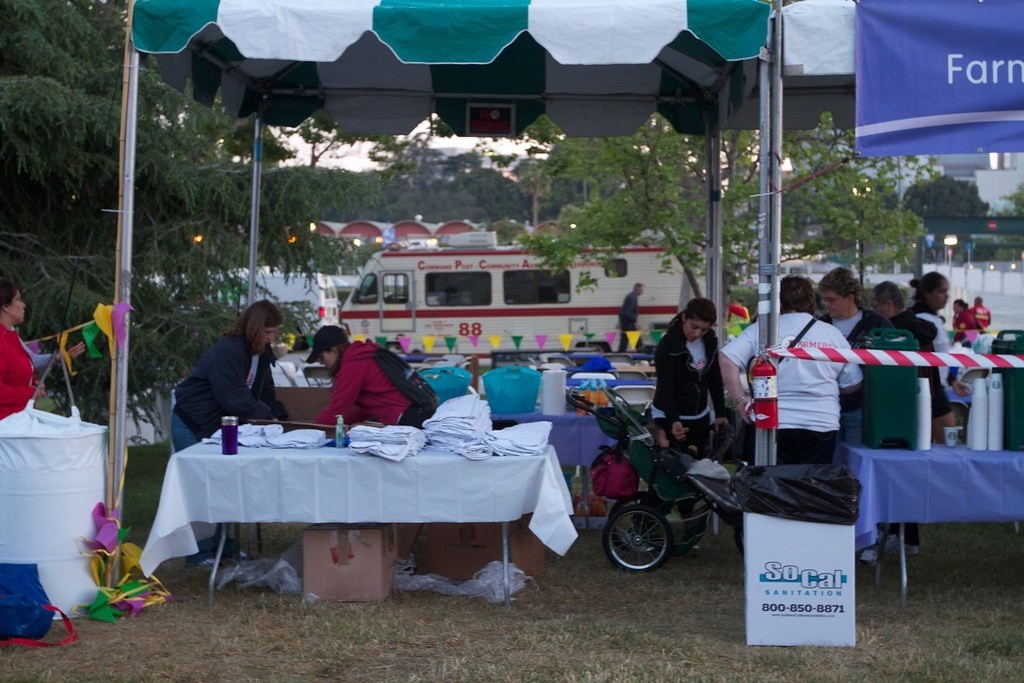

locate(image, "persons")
(817, 266), (895, 566)
(970, 297), (991, 328)
(616, 284), (644, 353)
(646, 425), (698, 471)
(907, 272), (972, 445)
(623, 297), (728, 551)
(171, 299), (290, 569)
(306, 325), (434, 577)
(725, 298), (750, 338)
(718, 275), (863, 465)
(0, 278), (66, 410)
(952, 299), (978, 342)
(868, 280), (937, 556)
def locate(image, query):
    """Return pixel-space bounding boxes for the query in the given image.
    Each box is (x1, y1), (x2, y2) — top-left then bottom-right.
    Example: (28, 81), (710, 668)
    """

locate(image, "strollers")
(570, 388), (862, 572)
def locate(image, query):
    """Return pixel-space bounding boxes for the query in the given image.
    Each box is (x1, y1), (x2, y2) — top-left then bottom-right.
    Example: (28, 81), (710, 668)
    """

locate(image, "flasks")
(221, 415), (238, 454)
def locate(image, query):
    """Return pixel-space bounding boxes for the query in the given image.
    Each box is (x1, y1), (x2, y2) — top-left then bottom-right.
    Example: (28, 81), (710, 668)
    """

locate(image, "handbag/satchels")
(416, 367), (473, 402)
(592, 442), (639, 500)
(482, 365), (543, 414)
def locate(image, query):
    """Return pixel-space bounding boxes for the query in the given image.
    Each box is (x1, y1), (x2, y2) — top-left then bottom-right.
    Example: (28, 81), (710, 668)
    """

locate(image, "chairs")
(418, 349), (666, 411)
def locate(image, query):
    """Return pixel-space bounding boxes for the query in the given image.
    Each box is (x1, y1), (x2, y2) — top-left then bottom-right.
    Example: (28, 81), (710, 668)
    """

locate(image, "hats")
(306, 325), (348, 363)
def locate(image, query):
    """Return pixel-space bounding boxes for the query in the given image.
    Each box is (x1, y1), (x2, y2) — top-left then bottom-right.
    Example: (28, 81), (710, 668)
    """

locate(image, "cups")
(943, 426), (959, 447)
(917, 377), (932, 450)
(970, 372), (1004, 451)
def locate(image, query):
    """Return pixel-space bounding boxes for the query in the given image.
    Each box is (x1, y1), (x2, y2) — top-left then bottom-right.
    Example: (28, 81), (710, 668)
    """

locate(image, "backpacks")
(373, 347), (439, 417)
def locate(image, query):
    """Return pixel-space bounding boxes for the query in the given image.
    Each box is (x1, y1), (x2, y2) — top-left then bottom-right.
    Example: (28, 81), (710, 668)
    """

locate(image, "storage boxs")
(296, 510), (555, 608)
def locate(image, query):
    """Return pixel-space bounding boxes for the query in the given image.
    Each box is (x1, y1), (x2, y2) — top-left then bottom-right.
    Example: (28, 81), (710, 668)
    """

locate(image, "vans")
(206, 266), (340, 338)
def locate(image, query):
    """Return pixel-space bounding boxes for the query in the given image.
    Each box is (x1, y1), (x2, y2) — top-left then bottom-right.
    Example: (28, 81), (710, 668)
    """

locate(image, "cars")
(780, 259), (843, 285)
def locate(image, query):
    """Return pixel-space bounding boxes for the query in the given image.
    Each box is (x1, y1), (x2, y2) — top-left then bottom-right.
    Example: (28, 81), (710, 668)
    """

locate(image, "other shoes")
(905, 544), (921, 555)
(885, 535), (899, 554)
(859, 543), (881, 564)
(622, 529), (655, 553)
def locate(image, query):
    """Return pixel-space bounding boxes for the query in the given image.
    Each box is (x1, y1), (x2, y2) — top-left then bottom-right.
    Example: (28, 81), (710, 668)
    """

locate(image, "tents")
(111, 0), (1024, 586)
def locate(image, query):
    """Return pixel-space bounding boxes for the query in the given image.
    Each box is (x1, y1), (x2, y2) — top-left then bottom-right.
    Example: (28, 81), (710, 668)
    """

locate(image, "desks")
(483, 413), (644, 530)
(614, 363), (656, 372)
(555, 351), (655, 359)
(835, 444), (1024, 595)
(139, 446), (577, 610)
(270, 377), (385, 420)
(565, 379), (654, 386)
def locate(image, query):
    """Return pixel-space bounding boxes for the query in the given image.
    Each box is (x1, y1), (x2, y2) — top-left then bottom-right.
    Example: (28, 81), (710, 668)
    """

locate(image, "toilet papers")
(542, 370), (566, 417)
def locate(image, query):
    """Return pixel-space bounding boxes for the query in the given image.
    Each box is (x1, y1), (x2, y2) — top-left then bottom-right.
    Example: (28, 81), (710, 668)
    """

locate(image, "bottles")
(576, 379), (608, 415)
(335, 415), (344, 448)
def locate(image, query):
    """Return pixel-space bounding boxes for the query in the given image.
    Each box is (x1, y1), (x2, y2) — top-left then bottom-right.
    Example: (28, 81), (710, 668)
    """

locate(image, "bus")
(336, 228), (708, 366)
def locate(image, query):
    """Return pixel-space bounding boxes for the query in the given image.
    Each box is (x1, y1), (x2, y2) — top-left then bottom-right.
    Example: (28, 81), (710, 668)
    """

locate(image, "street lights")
(944, 235), (959, 280)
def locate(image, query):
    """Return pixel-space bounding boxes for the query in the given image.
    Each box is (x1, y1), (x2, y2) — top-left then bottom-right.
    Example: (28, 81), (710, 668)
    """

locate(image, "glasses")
(822, 294), (845, 303)
